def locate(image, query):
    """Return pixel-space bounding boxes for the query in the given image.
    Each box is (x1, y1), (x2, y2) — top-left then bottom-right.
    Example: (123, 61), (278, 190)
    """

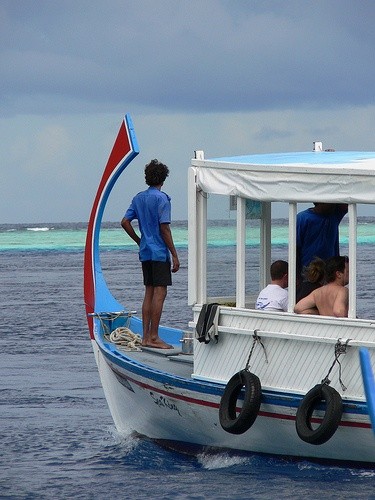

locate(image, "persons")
(255, 260), (288, 311)
(294, 256), (349, 318)
(121, 159), (180, 349)
(296, 202), (348, 287)
(296, 259), (327, 304)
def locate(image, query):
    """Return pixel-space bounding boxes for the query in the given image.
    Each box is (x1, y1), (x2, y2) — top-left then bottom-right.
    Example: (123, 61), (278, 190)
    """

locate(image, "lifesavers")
(219, 371), (262, 435)
(295, 384), (343, 446)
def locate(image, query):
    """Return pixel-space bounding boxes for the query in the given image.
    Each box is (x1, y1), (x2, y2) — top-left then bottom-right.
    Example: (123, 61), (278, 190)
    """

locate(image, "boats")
(83, 112), (375, 462)
